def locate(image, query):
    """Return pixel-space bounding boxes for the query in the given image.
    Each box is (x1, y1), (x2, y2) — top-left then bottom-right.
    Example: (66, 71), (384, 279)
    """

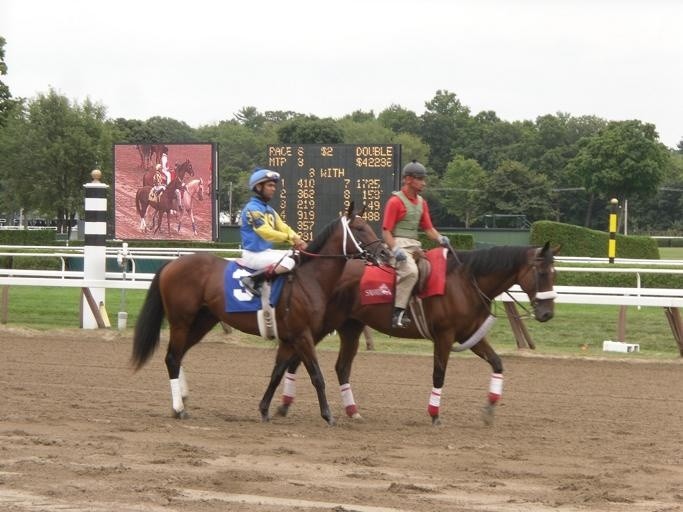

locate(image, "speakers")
(390, 306), (409, 330)
(239, 266), (277, 298)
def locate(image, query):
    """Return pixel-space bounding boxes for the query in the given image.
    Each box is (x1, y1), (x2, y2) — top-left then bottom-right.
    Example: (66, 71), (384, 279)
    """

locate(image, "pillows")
(399, 158), (428, 178)
(250, 170), (281, 190)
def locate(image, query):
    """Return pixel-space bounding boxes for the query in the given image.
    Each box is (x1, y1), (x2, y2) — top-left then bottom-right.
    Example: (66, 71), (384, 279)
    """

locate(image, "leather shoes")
(392, 246), (408, 260)
(436, 234), (450, 247)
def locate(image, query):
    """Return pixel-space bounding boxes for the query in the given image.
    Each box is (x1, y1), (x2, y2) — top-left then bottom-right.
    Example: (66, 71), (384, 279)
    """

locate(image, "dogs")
(137, 144), (168, 171)
(136, 159), (203, 236)
(130, 202), (392, 425)
(269, 240), (564, 430)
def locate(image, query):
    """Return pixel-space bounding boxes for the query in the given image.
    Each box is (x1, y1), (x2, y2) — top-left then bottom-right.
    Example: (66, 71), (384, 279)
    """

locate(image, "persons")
(159, 146), (172, 186)
(150, 162), (167, 197)
(238, 166), (307, 299)
(380, 157), (451, 332)
